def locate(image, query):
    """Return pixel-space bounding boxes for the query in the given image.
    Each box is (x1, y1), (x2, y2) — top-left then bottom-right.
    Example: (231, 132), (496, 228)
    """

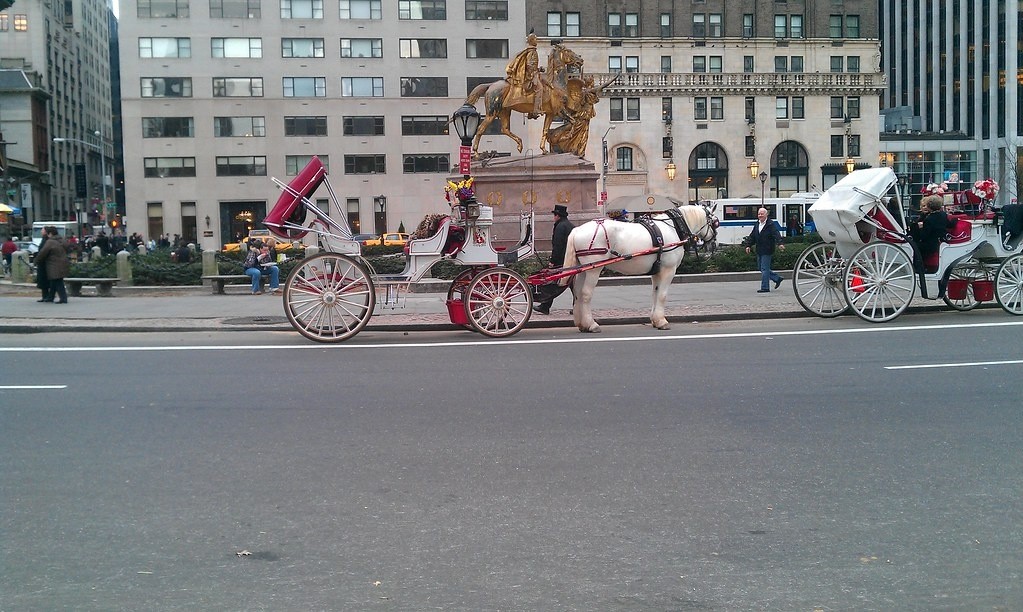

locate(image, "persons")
(916, 194), (948, 272)
(33, 226), (68, 304)
(886, 196), (910, 232)
(546, 70), (622, 159)
(504, 27), (545, 120)
(787, 215), (801, 237)
(532, 204), (577, 315)
(67, 231), (191, 262)
(744, 207), (785, 293)
(917, 197), (932, 222)
(2, 236), (17, 274)
(238, 237), (283, 296)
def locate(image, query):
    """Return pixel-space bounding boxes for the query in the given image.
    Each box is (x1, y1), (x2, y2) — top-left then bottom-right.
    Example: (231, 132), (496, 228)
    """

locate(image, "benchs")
(938, 190), (997, 223)
(401, 216), (451, 275)
(925, 217), (973, 265)
(314, 217), (361, 256)
(200, 275), (269, 295)
(858, 214), (915, 263)
(62, 277), (122, 296)
(452, 186), (494, 222)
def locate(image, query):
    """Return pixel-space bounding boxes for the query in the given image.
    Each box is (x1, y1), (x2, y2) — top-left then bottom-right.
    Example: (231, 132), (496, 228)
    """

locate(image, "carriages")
(263, 158), (719, 339)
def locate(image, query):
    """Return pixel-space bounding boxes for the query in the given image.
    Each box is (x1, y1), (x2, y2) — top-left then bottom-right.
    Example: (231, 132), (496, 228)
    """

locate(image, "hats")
(622, 210), (628, 214)
(551, 205), (568, 216)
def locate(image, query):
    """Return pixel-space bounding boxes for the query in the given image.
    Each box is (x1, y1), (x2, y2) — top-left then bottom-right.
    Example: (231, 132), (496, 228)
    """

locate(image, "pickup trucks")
(363, 233), (412, 246)
(225, 236), (293, 251)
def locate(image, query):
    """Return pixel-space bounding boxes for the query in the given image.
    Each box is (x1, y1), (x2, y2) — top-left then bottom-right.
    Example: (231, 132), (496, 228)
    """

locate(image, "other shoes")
(533, 305), (549, 315)
(775, 277), (783, 289)
(55, 299), (68, 304)
(269, 289), (283, 295)
(252, 291), (261, 295)
(37, 298), (54, 303)
(570, 310), (573, 315)
(757, 289), (770, 293)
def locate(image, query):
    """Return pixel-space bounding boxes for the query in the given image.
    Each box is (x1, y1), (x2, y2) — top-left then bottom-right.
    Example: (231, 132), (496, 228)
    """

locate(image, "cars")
(352, 234), (378, 246)
(13, 242), (39, 263)
(249, 230), (271, 236)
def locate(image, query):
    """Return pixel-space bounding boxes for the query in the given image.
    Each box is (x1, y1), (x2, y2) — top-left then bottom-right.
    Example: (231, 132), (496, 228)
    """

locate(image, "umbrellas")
(0, 203), (12, 214)
(4, 204), (20, 214)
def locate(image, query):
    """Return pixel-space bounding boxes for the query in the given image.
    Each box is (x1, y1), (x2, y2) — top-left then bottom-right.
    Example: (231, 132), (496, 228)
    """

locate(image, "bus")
(699, 193), (824, 247)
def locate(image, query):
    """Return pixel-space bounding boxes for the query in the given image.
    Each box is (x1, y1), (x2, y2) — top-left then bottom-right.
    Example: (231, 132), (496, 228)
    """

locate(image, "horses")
(557, 200), (719, 333)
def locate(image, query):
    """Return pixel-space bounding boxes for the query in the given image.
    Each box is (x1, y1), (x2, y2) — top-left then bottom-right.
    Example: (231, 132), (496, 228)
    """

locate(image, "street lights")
(759, 170), (767, 208)
(897, 171), (908, 207)
(73, 196), (83, 260)
(602, 125), (616, 217)
(453, 104), (482, 185)
(54, 138), (108, 225)
(378, 194), (386, 246)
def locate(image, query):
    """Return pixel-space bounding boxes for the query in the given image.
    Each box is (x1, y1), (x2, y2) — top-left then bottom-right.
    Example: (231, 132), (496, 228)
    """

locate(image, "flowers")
(920, 183), (947, 195)
(972, 178), (1000, 202)
(444, 177), (474, 204)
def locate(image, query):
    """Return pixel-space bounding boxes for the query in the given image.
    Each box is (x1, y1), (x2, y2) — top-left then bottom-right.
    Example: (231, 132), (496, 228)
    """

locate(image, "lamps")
(750, 156), (759, 179)
(665, 157), (676, 181)
(845, 154), (854, 174)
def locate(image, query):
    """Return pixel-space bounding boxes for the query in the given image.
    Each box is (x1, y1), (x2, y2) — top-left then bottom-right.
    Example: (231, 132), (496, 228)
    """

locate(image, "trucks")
(32, 222), (77, 247)
